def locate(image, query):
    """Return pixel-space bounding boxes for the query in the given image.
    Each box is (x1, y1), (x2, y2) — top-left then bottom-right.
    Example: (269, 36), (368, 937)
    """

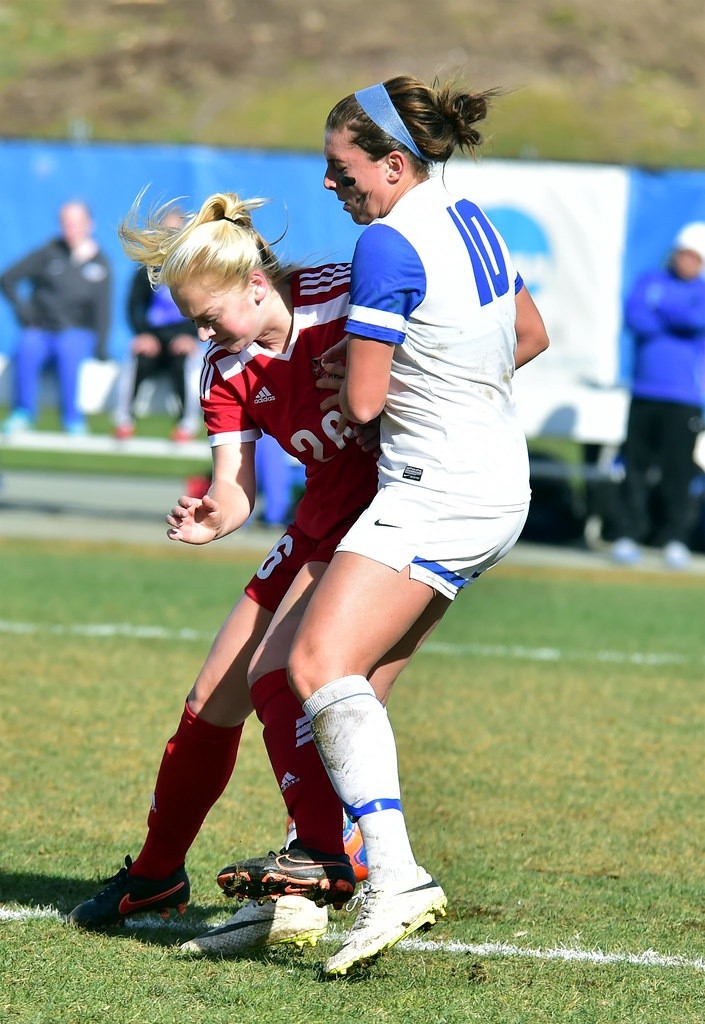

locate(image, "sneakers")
(180, 895), (329, 956)
(217, 839), (355, 910)
(67, 854), (190, 927)
(323, 866), (448, 974)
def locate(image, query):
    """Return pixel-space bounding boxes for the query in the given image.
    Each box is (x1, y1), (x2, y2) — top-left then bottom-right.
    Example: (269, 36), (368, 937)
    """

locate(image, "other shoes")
(610, 534), (635, 572)
(667, 539), (694, 569)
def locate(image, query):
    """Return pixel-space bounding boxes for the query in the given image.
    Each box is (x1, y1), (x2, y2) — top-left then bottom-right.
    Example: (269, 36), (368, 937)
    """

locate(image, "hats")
(679, 222), (705, 257)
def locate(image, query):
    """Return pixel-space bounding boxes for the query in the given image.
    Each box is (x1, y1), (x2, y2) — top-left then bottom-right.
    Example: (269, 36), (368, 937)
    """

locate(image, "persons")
(183, 75), (547, 978)
(73, 182), (378, 928)
(0, 200), (112, 443)
(111, 213), (205, 440)
(612, 222), (705, 573)
